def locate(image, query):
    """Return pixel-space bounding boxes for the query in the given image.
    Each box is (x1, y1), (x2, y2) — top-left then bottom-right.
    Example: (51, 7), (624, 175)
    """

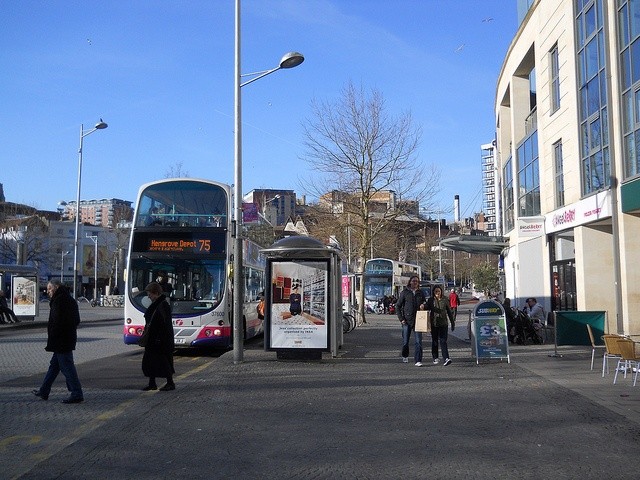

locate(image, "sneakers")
(403, 357), (409, 362)
(444, 358), (452, 366)
(414, 361), (424, 366)
(433, 359), (440, 365)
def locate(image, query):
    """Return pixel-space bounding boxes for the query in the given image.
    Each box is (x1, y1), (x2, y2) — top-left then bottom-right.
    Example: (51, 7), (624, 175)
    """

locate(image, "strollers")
(516, 310), (543, 345)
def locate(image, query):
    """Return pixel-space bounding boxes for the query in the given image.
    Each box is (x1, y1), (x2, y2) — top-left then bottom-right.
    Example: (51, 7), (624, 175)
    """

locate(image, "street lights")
(61, 250), (71, 283)
(234, 51), (305, 363)
(74, 118), (107, 302)
(264, 194), (280, 218)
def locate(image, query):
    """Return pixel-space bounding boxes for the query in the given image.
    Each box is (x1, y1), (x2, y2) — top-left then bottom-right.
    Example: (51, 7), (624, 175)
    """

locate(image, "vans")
(472, 282), (488, 299)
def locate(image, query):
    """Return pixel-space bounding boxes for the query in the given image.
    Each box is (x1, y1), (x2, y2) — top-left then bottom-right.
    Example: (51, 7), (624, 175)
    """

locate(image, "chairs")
(612, 339), (640, 387)
(586, 324), (606, 371)
(541, 312), (554, 340)
(601, 334), (632, 378)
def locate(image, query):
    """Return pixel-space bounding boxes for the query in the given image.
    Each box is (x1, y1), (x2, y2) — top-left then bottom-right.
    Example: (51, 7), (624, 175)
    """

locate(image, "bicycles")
(342, 302), (350, 331)
(344, 315), (356, 332)
(104, 294), (120, 308)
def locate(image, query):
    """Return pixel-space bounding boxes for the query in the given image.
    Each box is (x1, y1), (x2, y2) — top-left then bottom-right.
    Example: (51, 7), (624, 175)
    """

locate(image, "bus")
(123, 179), (274, 347)
(363, 258), (421, 310)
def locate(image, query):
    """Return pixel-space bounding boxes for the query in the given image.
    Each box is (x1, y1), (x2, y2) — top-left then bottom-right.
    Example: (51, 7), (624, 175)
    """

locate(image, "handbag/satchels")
(415, 306), (431, 333)
(139, 324), (149, 347)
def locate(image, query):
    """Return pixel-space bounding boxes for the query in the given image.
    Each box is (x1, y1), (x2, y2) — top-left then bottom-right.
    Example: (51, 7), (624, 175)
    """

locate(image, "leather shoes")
(160, 384), (175, 391)
(142, 385), (157, 390)
(32, 390), (48, 400)
(62, 391), (84, 403)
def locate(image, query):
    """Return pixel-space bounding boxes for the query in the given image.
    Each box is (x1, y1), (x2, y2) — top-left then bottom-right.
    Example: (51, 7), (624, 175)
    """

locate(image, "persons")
(397, 275), (427, 366)
(449, 289), (460, 321)
(423, 285), (455, 366)
(0, 290), (21, 323)
(32, 280), (85, 403)
(143, 281), (176, 390)
(496, 298), (545, 343)
(211, 210), (225, 227)
(143, 205), (159, 226)
(289, 288), (301, 315)
